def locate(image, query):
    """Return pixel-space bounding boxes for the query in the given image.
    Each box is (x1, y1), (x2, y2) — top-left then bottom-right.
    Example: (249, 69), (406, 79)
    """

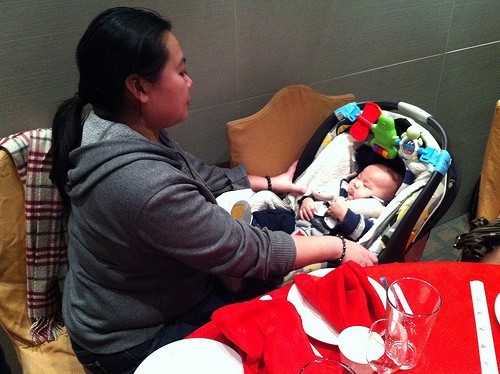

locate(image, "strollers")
(214, 99), (462, 303)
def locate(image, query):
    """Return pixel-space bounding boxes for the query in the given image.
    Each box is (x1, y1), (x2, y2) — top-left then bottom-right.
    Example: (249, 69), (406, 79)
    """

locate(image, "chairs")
(226, 85), (354, 177)
(0, 129), (92, 374)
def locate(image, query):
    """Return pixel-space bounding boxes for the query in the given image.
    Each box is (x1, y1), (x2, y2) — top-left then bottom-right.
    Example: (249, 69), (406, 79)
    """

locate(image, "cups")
(383, 277), (444, 371)
(338, 325), (385, 374)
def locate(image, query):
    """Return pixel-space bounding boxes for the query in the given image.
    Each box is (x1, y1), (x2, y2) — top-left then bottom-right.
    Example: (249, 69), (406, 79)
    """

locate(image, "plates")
(286, 267), (398, 346)
(132, 337), (245, 374)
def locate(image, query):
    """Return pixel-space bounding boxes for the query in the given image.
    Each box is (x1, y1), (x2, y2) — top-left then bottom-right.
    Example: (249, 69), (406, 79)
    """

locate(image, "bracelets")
(265, 175), (272, 191)
(321, 231), (346, 263)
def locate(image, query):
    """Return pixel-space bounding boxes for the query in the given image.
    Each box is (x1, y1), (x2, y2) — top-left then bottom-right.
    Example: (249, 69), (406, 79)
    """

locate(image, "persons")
(48, 5), (379, 374)
(232, 161), (403, 287)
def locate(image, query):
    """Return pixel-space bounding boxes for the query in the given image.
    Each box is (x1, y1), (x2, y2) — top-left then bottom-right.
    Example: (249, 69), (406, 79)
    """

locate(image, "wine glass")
(363, 319), (408, 374)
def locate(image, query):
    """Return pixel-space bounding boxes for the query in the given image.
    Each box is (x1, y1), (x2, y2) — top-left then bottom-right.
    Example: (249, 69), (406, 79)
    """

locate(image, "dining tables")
(186, 263), (500, 374)
